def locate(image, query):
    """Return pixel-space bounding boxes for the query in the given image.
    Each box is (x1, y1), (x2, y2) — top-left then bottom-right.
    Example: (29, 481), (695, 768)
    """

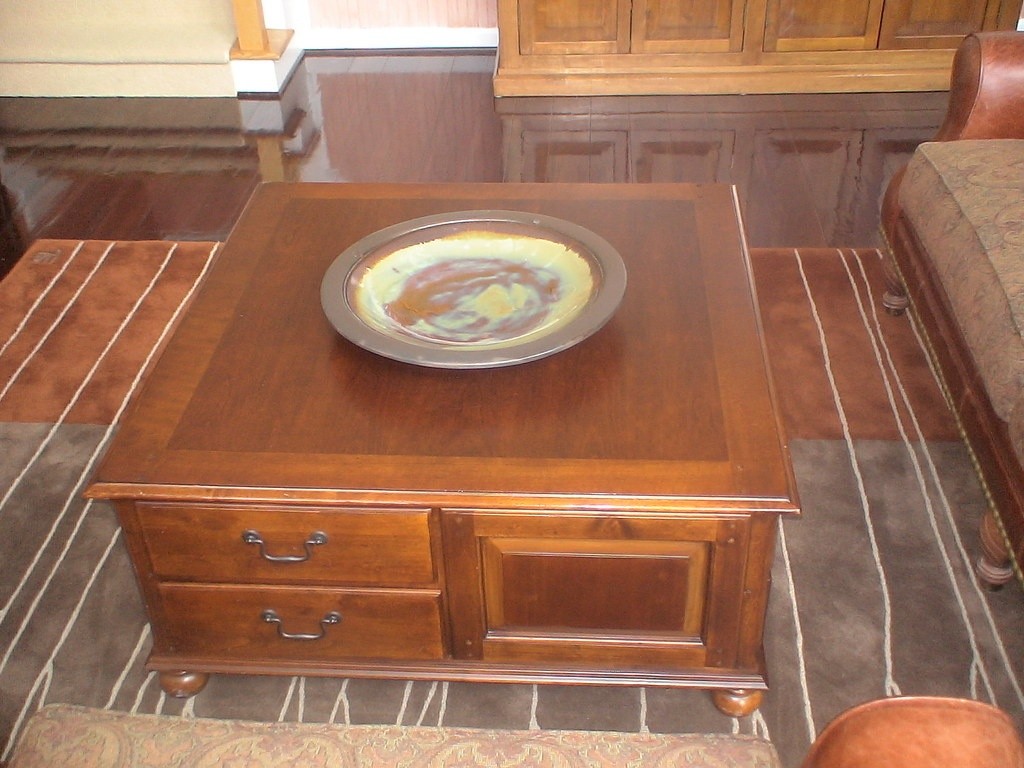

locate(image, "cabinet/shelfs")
(493, 92), (953, 248)
(492, 1), (1024, 96)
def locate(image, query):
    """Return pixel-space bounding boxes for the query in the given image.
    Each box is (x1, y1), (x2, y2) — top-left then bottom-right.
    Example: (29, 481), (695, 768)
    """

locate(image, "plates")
(319, 209), (627, 369)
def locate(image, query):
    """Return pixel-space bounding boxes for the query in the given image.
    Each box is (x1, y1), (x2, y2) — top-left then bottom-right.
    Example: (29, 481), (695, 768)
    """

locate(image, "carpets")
(0, 240), (1023, 768)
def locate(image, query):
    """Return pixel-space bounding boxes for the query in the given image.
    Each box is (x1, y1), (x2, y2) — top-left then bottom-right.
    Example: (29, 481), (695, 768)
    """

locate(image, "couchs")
(10, 697), (1024, 768)
(879, 32), (1023, 592)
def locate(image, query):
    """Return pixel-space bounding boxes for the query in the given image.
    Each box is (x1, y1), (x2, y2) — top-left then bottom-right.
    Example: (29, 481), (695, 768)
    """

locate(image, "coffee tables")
(84, 182), (798, 718)
